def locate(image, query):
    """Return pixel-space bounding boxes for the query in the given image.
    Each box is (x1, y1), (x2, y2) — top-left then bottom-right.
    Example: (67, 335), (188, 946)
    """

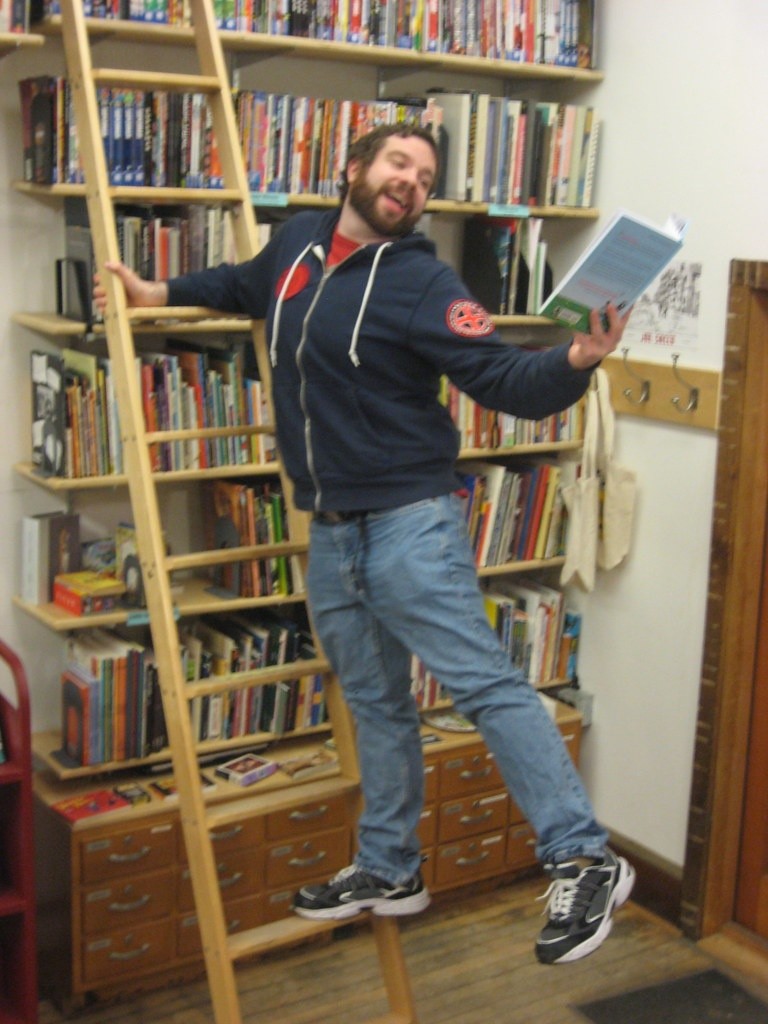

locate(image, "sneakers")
(288, 864), (432, 920)
(536, 846), (637, 966)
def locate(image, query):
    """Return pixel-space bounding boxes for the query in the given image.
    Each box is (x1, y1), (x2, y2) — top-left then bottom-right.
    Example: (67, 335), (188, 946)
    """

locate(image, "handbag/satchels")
(557, 390), (598, 593)
(592, 365), (636, 570)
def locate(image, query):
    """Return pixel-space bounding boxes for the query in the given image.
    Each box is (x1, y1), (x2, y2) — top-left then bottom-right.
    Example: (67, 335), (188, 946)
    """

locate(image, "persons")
(92, 125), (635, 965)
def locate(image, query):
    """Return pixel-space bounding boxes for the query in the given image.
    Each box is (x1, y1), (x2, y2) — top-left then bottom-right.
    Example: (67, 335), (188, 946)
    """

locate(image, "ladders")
(58, 0), (421, 1024)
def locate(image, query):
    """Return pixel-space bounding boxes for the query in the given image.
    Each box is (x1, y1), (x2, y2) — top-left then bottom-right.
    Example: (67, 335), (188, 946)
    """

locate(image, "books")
(460, 217), (549, 318)
(58, 197), (284, 323)
(31, 337), (279, 477)
(207, 480), (296, 596)
(49, 790), (128, 826)
(118, 782), (149, 806)
(215, 753), (280, 787)
(0, 0), (598, 69)
(483, 577), (580, 682)
(61, 605), (326, 766)
(24, 510), (170, 615)
(409, 652), (453, 707)
(540, 211), (682, 331)
(325, 737), (338, 750)
(148, 771), (219, 802)
(458, 456), (570, 566)
(439, 375), (584, 450)
(17, 79), (599, 204)
(276, 748), (341, 779)
